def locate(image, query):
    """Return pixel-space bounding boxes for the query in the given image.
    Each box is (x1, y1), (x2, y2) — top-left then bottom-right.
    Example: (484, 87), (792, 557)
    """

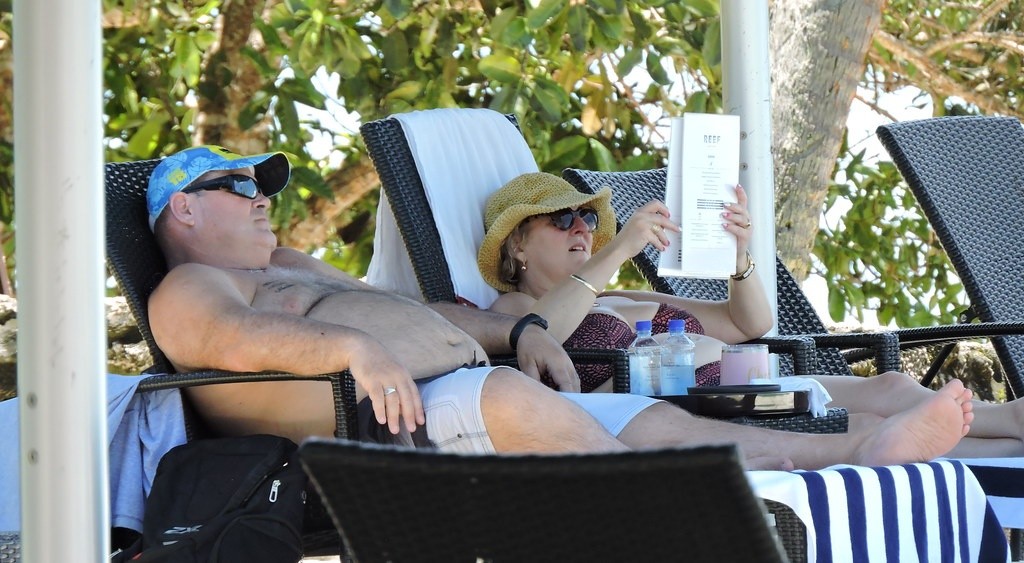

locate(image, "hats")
(146, 144), (290, 234)
(478, 173), (616, 292)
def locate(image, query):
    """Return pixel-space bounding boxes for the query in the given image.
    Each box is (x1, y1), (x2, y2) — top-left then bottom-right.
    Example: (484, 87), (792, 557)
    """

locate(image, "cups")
(769, 353), (779, 385)
(721, 343), (769, 385)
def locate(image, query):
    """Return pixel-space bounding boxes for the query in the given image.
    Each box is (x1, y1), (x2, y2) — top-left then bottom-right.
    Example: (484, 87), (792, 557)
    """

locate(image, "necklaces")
(533, 295), (600, 307)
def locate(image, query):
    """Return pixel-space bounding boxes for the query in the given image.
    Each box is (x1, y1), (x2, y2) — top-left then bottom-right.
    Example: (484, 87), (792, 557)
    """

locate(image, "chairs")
(103, 109), (1024, 563)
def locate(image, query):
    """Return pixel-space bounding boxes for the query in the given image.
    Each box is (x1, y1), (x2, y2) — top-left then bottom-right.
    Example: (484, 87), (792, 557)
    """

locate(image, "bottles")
(661, 320), (696, 397)
(627, 320), (661, 398)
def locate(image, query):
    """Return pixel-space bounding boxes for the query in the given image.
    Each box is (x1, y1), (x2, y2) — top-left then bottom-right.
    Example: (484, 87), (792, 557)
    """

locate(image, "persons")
(145, 143), (974, 467)
(476, 171), (1024, 458)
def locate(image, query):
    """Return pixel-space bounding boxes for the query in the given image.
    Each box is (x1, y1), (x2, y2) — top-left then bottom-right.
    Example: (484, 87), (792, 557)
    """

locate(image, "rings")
(385, 387), (395, 395)
(652, 225), (661, 233)
(744, 219), (751, 228)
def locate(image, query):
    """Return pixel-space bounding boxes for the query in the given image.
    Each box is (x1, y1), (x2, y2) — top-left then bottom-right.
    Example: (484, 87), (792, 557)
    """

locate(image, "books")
(657, 112), (740, 281)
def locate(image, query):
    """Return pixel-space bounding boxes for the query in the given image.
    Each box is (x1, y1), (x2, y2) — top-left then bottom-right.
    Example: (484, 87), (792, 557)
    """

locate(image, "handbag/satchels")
(117, 432), (305, 563)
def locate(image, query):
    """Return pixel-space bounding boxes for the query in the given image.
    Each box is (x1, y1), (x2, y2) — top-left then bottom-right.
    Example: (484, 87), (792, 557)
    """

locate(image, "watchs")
(509, 313), (547, 351)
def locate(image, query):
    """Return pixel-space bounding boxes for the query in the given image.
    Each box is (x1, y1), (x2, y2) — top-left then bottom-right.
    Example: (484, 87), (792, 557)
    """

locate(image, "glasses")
(524, 208), (599, 233)
(183, 174), (267, 199)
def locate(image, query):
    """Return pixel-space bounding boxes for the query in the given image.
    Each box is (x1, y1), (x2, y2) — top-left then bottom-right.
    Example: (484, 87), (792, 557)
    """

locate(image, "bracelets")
(731, 250), (756, 281)
(570, 274), (598, 298)
